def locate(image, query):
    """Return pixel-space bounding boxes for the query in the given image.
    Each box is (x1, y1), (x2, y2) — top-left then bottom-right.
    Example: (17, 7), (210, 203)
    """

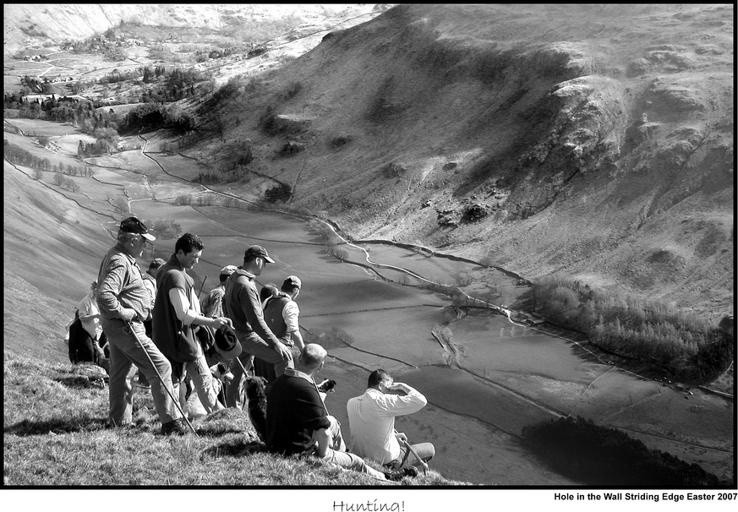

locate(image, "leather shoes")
(161, 419), (187, 435)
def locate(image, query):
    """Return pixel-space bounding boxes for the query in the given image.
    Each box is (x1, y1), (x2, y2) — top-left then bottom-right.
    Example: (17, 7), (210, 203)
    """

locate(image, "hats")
(285, 275), (302, 289)
(220, 264), (237, 275)
(245, 245), (275, 263)
(120, 217), (156, 241)
(214, 324), (242, 360)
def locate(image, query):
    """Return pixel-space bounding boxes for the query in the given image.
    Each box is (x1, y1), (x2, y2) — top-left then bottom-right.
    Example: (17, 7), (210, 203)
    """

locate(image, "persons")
(98, 217), (197, 436)
(264, 343), (418, 483)
(223, 245), (295, 409)
(346, 368), (435, 478)
(155, 232), (237, 415)
(81, 257), (305, 378)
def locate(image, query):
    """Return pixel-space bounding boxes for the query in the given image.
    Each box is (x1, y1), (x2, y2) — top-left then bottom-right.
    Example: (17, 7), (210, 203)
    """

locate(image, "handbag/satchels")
(69, 319), (94, 363)
